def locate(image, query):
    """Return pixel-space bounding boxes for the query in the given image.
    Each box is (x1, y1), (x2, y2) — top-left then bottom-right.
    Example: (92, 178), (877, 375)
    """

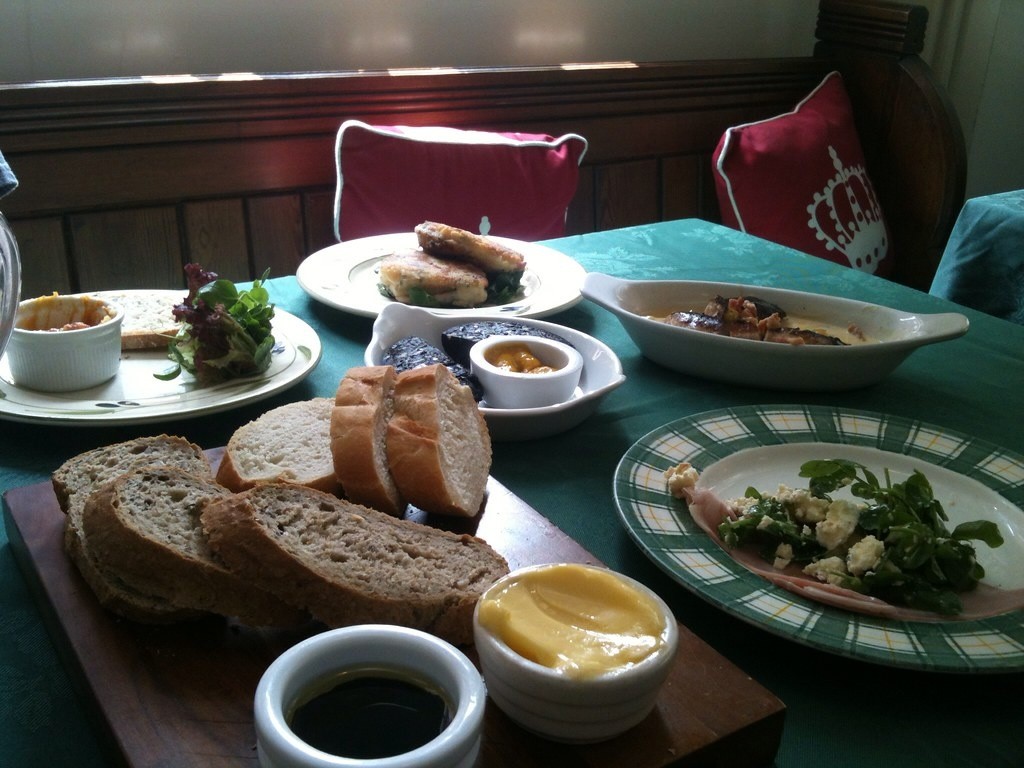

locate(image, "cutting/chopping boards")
(2, 444), (788, 768)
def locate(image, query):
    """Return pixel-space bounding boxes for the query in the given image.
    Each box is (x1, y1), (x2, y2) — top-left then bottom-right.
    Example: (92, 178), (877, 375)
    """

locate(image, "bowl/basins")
(6, 294), (125, 392)
(474, 563), (680, 744)
(255, 623), (485, 768)
(468, 336), (583, 408)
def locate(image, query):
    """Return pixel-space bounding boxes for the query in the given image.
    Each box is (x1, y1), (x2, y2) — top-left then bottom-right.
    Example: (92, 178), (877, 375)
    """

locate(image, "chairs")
(887, 53), (973, 297)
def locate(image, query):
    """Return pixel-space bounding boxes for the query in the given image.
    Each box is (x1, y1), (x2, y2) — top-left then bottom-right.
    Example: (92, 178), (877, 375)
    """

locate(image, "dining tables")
(1, 205), (1024, 767)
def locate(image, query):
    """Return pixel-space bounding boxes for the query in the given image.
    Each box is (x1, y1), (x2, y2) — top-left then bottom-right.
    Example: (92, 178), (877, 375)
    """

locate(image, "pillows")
(711, 69), (896, 287)
(329, 117), (590, 253)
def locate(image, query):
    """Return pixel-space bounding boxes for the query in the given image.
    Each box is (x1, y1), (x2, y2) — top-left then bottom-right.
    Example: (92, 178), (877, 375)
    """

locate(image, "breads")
(47, 356), (513, 653)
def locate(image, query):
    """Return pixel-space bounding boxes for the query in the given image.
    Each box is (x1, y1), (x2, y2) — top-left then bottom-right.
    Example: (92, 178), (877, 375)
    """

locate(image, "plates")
(295, 232), (590, 319)
(580, 271), (969, 393)
(364, 303), (626, 441)
(1, 289), (324, 426)
(614, 405), (1023, 673)
